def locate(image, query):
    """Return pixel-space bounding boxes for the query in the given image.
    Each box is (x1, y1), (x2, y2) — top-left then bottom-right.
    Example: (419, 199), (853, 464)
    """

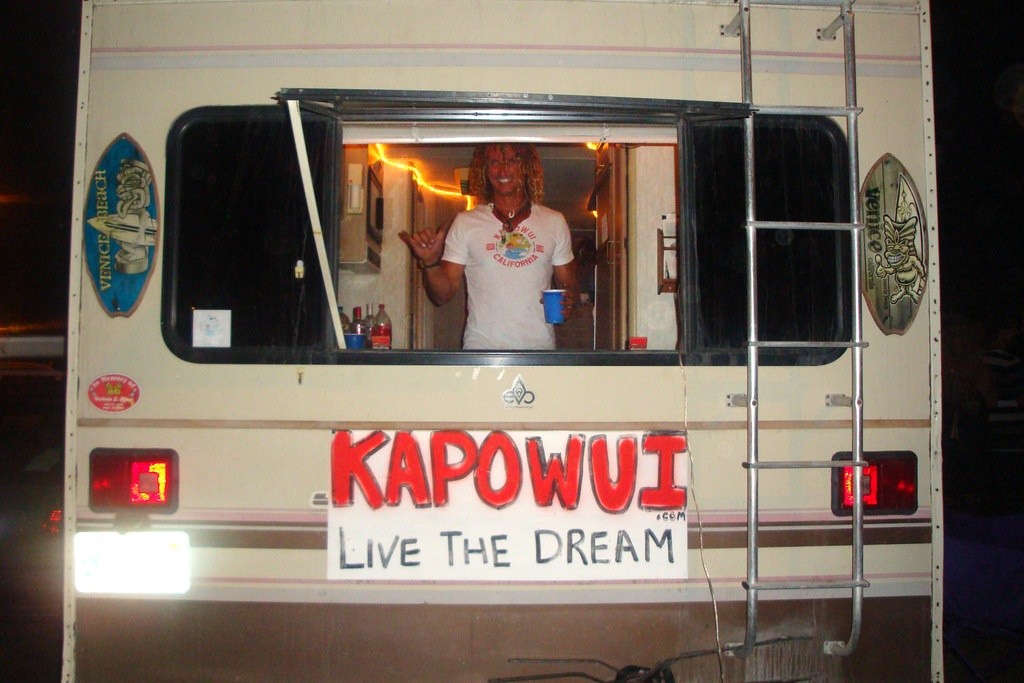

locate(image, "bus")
(62, 1), (944, 683)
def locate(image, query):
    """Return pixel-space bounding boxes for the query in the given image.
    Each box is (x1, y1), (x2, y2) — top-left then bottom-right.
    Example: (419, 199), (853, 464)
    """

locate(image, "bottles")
(338, 306), (352, 333)
(376, 304), (393, 348)
(351, 307), (365, 334)
(365, 304), (377, 348)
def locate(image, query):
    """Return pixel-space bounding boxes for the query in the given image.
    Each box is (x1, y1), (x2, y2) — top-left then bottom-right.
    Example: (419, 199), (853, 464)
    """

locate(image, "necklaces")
(492, 199), (529, 233)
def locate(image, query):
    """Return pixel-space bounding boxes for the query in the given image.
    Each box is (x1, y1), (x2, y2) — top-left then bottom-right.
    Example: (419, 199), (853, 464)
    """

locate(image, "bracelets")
(423, 256), (440, 268)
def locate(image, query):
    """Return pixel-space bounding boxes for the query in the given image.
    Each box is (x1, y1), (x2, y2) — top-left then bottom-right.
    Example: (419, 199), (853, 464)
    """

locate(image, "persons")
(400, 143), (579, 350)
(572, 236), (595, 302)
(942, 298), (1024, 513)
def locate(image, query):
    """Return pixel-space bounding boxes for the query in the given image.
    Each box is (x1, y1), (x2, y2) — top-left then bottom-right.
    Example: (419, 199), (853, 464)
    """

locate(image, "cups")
(541, 289), (566, 324)
(345, 333), (366, 350)
